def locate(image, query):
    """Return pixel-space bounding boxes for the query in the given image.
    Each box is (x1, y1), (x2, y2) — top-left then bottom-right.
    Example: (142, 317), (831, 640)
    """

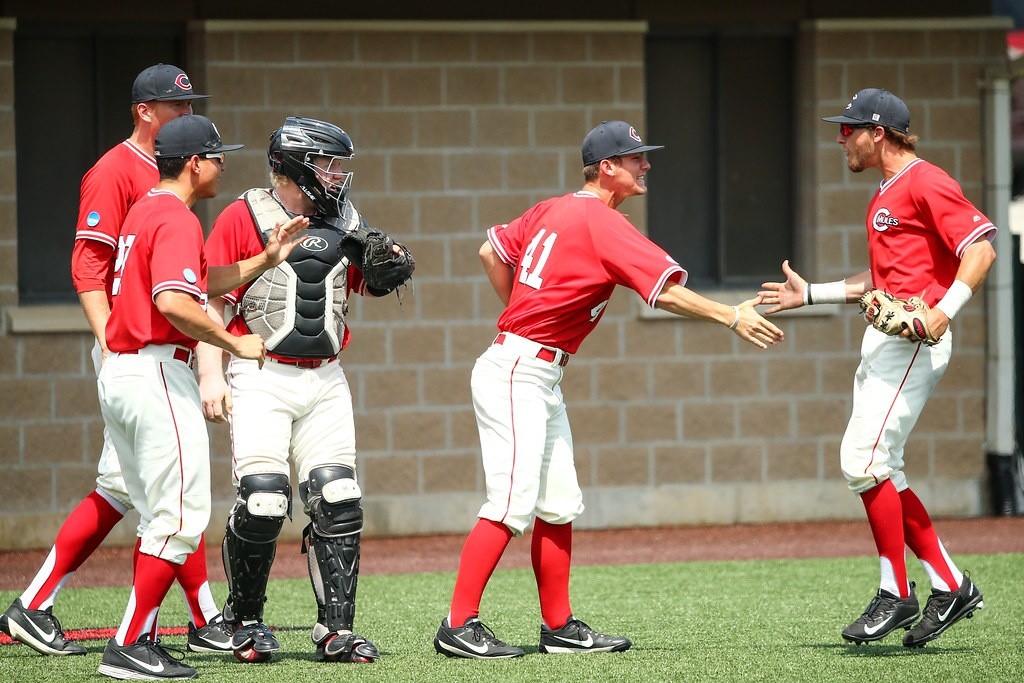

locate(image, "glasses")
(197, 151), (226, 166)
(840, 123), (876, 137)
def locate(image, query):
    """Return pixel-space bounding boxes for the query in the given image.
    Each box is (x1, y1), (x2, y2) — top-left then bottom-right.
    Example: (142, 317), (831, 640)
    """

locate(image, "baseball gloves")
(859, 286), (941, 348)
(337, 225), (416, 298)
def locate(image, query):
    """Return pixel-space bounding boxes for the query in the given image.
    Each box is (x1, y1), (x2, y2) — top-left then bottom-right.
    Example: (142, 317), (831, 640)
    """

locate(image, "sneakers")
(187, 613), (234, 653)
(0, 598), (88, 657)
(98, 634), (200, 681)
(233, 621), (271, 663)
(842, 581), (920, 647)
(539, 615), (631, 654)
(317, 633), (375, 663)
(901, 569), (985, 649)
(433, 617), (524, 660)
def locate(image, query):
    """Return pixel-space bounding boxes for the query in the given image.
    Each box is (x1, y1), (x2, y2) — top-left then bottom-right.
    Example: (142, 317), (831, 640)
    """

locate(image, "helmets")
(269, 117), (354, 221)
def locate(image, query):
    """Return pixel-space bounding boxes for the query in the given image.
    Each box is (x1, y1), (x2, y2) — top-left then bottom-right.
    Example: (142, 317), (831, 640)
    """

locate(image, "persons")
(434, 121), (784, 661)
(97, 113), (309, 683)
(1, 62), (237, 656)
(755, 89), (999, 649)
(197, 115), (418, 662)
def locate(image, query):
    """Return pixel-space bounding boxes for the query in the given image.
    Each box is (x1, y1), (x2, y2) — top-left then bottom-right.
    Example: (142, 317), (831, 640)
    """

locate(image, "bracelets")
(808, 283), (814, 305)
(729, 306), (739, 328)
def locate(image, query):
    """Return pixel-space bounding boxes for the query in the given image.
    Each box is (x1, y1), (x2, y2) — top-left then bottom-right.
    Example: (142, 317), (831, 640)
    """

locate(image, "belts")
(495, 333), (568, 369)
(279, 354), (338, 369)
(119, 343), (193, 369)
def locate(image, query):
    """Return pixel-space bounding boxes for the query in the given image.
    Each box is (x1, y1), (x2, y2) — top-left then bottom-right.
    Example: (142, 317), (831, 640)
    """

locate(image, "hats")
(153, 115), (245, 158)
(823, 87), (910, 135)
(131, 63), (212, 103)
(582, 120), (664, 168)
